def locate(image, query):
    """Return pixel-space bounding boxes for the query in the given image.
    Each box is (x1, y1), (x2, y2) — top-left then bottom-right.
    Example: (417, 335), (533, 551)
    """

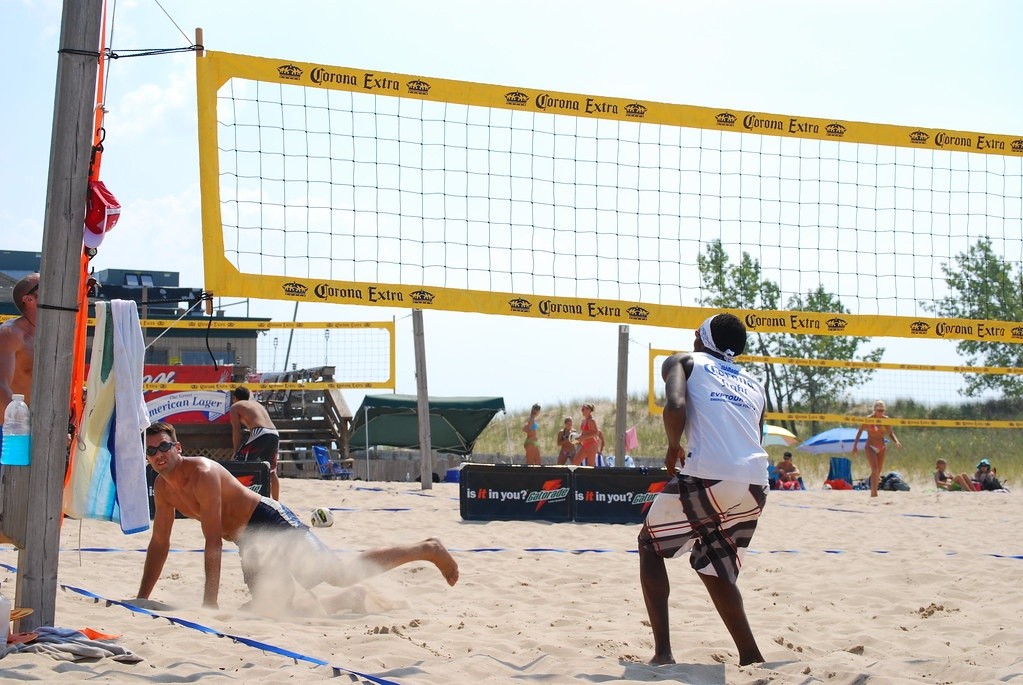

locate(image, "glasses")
(146, 441), (177, 457)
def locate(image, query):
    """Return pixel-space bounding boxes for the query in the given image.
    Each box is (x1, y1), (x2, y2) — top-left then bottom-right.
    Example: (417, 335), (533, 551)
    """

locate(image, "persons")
(0, 273), (72, 545)
(851, 400), (902, 497)
(766, 452), (800, 490)
(934, 458), (1003, 491)
(230, 386), (280, 500)
(522, 403), (605, 466)
(137, 422), (460, 616)
(638, 313), (770, 667)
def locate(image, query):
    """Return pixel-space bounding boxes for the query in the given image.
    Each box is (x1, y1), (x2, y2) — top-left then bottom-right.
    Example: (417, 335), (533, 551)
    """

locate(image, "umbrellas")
(796, 424), (889, 479)
(761, 420), (798, 451)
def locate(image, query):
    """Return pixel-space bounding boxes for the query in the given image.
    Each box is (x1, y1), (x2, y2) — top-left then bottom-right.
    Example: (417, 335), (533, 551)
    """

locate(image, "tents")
(348, 394), (513, 482)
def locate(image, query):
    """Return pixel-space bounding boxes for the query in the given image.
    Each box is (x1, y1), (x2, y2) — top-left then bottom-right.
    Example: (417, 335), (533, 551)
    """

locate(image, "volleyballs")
(311, 507), (334, 528)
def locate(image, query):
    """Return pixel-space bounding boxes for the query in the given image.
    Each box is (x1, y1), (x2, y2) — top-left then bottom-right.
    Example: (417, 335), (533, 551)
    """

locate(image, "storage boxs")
(446, 466), (460, 483)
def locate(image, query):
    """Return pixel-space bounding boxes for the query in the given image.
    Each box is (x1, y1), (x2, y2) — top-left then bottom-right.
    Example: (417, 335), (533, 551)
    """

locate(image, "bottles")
(0, 393), (30, 465)
(0, 583), (12, 659)
(406, 472), (412, 482)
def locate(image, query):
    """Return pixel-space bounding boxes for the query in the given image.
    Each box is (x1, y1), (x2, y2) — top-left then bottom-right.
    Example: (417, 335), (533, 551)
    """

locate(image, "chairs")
(312, 445), (355, 481)
(581, 453), (635, 467)
(932, 471), (962, 491)
